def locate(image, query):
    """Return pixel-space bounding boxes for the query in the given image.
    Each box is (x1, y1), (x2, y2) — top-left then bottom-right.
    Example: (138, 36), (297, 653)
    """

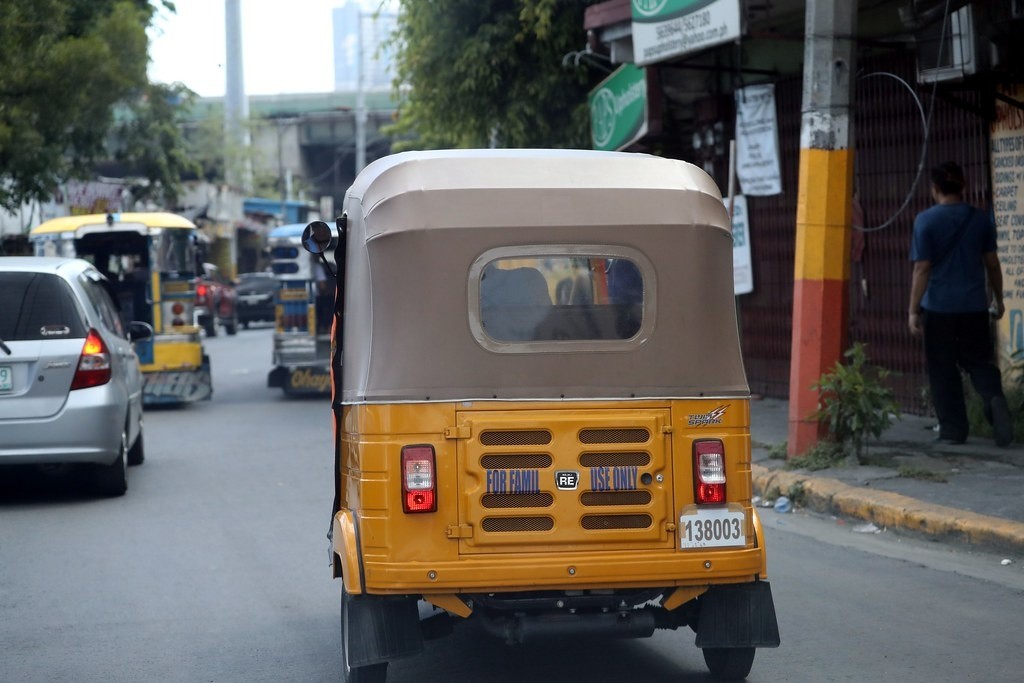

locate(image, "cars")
(230, 271), (280, 330)
(0, 253), (157, 500)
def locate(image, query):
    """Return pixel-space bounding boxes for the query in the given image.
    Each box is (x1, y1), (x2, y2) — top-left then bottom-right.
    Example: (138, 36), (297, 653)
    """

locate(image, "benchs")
(483, 304), (637, 340)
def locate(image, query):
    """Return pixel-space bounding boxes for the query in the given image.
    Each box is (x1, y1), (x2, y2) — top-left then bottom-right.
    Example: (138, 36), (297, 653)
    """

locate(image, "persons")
(908, 161), (1016, 448)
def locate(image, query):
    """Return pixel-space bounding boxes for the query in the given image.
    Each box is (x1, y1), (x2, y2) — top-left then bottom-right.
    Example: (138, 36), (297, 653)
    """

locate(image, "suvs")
(195, 260), (239, 339)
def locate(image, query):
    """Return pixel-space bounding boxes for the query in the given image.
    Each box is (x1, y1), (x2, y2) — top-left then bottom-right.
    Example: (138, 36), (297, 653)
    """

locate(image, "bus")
(257, 219), (339, 330)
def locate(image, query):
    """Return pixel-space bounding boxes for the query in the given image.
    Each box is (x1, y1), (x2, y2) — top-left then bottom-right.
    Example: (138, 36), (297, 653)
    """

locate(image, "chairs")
(492, 267), (553, 304)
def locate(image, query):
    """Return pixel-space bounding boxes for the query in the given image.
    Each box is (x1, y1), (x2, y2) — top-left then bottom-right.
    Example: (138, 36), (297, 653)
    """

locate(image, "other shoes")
(934, 437), (964, 446)
(991, 396), (1014, 447)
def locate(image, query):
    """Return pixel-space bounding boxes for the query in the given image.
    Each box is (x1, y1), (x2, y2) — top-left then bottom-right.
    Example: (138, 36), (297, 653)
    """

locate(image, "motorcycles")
(299, 146), (782, 682)
